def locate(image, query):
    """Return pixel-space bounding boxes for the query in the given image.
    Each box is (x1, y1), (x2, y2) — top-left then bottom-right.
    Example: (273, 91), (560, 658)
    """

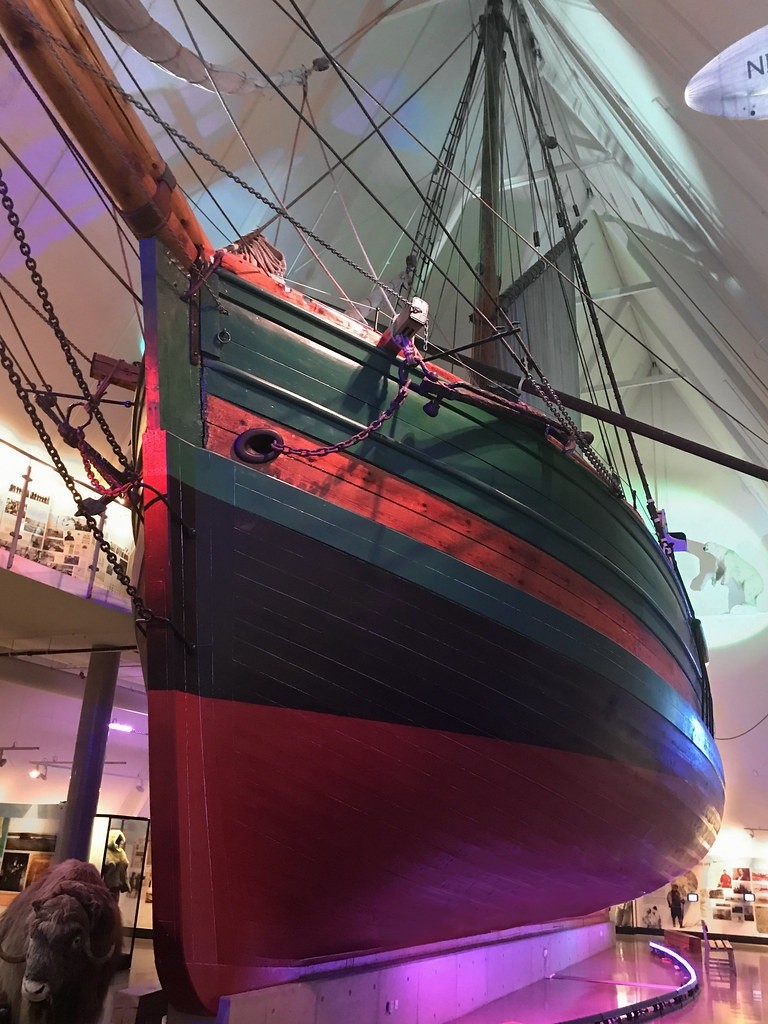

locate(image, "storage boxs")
(88, 814), (150, 969)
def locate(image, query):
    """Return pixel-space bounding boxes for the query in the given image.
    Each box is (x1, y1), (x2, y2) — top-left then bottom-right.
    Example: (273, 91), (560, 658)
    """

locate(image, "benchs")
(707, 970), (737, 1023)
(701, 919), (737, 977)
(664, 929), (701, 953)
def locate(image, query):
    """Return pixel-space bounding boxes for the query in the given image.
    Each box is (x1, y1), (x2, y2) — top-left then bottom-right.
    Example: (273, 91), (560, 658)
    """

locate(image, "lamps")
(40, 765), (48, 781)
(0, 749), (7, 767)
(136, 778), (145, 793)
(749, 830), (754, 838)
(29, 764), (40, 779)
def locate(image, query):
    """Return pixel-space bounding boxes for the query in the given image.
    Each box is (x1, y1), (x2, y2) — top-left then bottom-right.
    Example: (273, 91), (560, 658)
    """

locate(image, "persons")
(666, 883), (687, 928)
(103, 828), (141, 907)
(735, 868), (750, 881)
(642, 905), (662, 928)
(720, 869), (732, 888)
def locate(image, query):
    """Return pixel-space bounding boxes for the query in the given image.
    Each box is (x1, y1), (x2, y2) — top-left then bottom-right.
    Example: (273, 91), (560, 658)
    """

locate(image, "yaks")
(0, 858), (123, 1024)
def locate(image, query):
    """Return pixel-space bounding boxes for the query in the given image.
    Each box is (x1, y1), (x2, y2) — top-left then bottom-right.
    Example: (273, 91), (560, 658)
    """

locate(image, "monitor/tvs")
(744, 893), (755, 902)
(687, 894), (698, 902)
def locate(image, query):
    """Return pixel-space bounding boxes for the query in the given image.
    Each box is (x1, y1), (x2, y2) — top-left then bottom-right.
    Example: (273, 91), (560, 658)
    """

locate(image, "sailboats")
(0, 0), (768, 1024)
